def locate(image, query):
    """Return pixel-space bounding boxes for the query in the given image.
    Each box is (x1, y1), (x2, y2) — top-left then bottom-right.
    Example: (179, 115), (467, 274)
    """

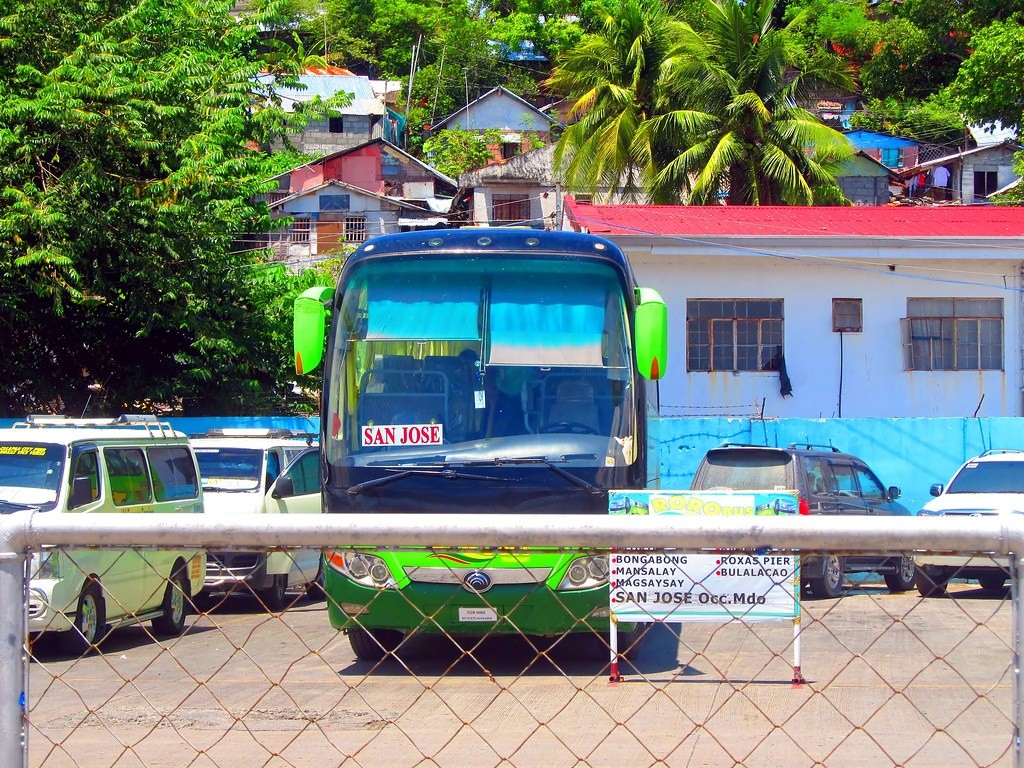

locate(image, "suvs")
(687, 442), (916, 599)
(916, 449), (1024, 598)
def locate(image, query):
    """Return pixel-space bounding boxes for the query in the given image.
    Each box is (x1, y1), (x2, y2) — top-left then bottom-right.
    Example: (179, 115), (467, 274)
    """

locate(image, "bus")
(293, 228), (666, 662)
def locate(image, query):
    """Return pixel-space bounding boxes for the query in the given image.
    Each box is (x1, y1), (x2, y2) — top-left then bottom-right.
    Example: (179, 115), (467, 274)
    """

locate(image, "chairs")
(815, 478), (839, 495)
(368, 352), (602, 440)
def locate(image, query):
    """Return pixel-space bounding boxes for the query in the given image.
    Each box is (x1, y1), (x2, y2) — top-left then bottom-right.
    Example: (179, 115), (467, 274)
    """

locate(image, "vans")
(2, 415), (323, 654)
